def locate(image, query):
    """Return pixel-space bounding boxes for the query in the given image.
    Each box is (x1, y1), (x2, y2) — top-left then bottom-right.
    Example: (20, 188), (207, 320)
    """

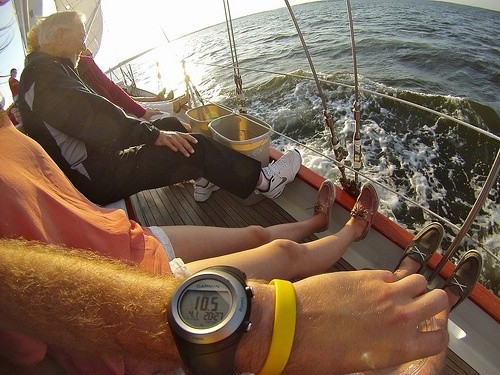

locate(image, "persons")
(0, 10), (482, 375)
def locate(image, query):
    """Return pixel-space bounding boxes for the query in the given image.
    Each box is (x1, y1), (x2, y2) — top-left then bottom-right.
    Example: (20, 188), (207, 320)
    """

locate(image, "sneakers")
(193, 180), (221, 202)
(256, 148), (301, 200)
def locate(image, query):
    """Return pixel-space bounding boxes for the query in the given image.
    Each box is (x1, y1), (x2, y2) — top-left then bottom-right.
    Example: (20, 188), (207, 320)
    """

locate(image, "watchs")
(166, 265), (254, 375)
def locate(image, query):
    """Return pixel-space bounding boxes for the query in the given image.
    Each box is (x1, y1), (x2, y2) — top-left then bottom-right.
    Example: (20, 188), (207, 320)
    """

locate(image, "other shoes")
(157, 87), (166, 101)
(175, 93), (190, 113)
(167, 90), (174, 100)
(394, 222), (445, 276)
(442, 249), (482, 311)
(306, 180), (336, 233)
(349, 183), (379, 242)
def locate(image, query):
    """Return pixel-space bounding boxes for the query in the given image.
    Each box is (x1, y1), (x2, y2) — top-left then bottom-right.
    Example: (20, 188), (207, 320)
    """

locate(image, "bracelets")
(257, 279), (296, 375)
